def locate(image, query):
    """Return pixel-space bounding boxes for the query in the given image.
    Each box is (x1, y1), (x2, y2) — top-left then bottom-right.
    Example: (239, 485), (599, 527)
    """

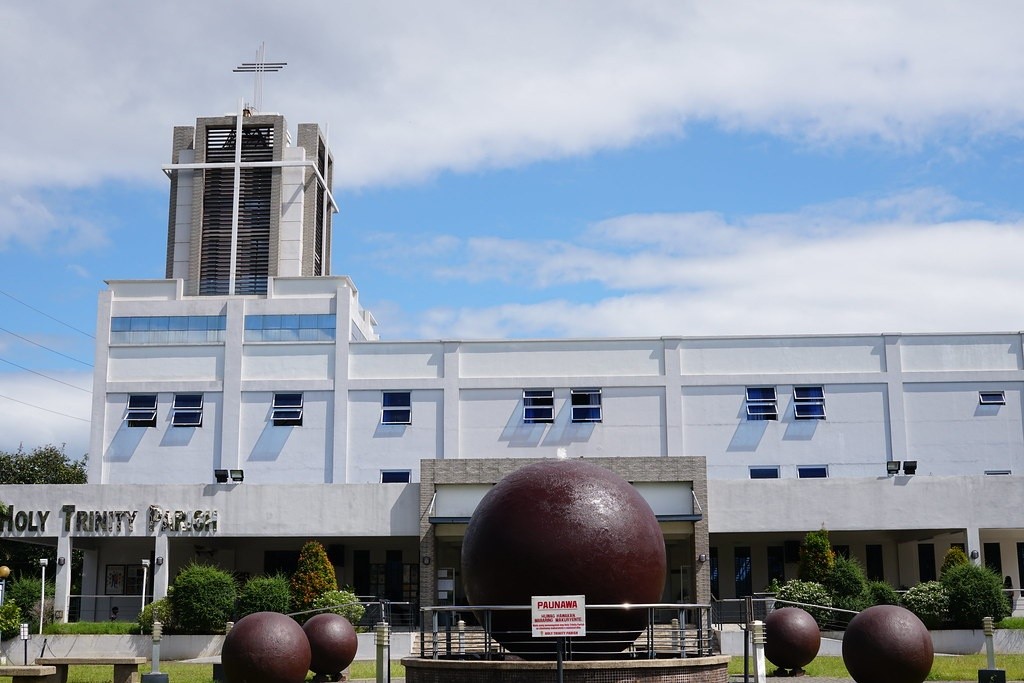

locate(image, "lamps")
(214, 469), (229, 484)
(903, 460), (917, 475)
(887, 461), (901, 474)
(230, 469), (244, 483)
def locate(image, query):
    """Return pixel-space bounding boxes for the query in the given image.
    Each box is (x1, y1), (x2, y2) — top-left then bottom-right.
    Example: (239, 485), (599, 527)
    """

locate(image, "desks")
(35, 657), (147, 683)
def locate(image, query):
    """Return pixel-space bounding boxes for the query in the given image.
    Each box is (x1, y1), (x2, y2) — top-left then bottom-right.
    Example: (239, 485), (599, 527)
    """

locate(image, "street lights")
(140, 558), (151, 635)
(38, 558), (48, 634)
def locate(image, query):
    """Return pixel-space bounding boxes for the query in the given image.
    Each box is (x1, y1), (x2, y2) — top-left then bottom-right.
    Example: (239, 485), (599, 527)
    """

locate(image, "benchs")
(0, 665), (56, 683)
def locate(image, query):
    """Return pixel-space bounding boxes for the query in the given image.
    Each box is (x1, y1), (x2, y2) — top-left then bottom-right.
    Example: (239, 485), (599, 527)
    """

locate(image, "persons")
(1002, 576), (1014, 618)
